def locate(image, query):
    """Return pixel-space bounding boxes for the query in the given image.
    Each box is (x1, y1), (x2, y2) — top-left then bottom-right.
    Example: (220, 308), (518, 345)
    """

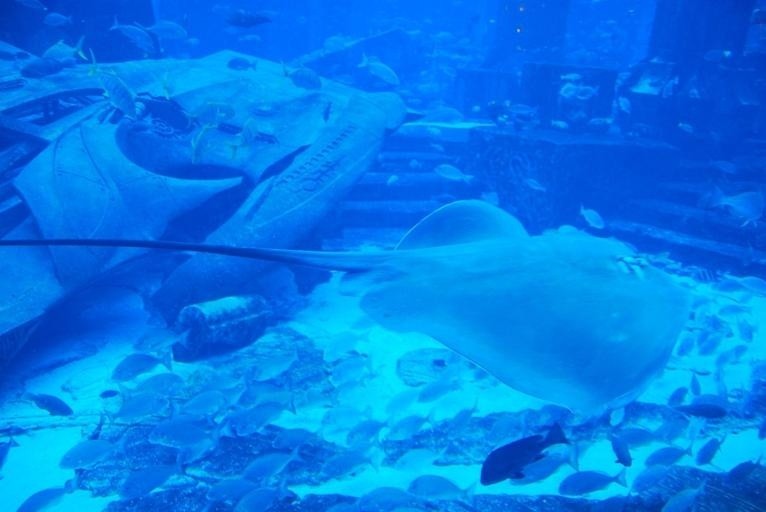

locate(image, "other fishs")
(0, 0), (765, 511)
